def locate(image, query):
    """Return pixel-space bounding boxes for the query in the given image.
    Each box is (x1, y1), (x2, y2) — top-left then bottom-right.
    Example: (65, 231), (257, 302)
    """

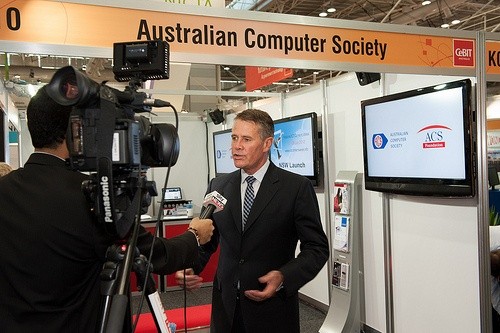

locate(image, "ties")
(242, 175), (256, 229)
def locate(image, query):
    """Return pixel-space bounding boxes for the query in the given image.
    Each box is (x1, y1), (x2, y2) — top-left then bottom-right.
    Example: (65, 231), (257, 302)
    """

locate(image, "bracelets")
(185, 227), (200, 244)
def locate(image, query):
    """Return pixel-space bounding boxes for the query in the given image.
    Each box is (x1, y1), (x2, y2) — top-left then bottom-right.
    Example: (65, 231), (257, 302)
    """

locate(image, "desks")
(130, 213), (221, 296)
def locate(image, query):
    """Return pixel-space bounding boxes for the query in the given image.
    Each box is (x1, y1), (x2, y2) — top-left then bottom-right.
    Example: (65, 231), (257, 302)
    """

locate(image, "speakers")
(355, 72), (381, 86)
(209, 108), (225, 125)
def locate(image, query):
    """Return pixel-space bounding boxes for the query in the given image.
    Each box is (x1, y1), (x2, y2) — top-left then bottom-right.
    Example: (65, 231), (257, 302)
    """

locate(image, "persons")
(0, 84), (214, 333)
(274, 136), (281, 160)
(176, 109), (330, 333)
(334, 188), (343, 212)
(0, 162), (13, 177)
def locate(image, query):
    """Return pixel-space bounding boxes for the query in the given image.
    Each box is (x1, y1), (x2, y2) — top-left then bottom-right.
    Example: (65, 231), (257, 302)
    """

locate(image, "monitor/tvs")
(269, 112), (321, 188)
(361, 79), (475, 197)
(213, 129), (239, 176)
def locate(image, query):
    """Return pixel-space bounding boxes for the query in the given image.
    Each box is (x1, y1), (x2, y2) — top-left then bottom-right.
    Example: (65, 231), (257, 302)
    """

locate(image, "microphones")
(199, 189), (227, 220)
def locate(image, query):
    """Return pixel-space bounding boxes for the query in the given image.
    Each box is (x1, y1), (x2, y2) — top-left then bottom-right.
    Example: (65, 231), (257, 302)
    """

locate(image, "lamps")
(421, 0), (432, 6)
(221, 65), (231, 71)
(326, 0), (336, 13)
(318, 7), (328, 16)
(312, 70), (320, 75)
(450, 16), (461, 24)
(441, 18), (450, 28)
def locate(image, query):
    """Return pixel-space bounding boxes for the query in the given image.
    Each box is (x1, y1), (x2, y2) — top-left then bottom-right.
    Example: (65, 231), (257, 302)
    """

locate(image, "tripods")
(94, 241), (172, 333)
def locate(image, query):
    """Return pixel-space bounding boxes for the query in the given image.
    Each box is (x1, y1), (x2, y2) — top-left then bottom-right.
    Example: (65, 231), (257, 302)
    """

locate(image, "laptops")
(162, 187), (185, 202)
